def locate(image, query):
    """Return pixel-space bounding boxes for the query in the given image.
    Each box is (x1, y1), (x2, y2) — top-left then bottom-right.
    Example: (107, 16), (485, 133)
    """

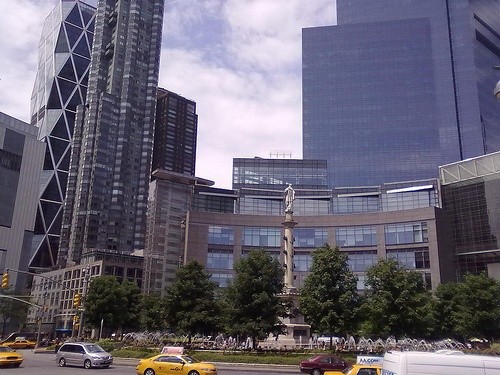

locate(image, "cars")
(135, 347), (218, 375)
(323, 354), (383, 375)
(0, 344), (25, 368)
(299, 353), (355, 375)
(0, 336), (36, 349)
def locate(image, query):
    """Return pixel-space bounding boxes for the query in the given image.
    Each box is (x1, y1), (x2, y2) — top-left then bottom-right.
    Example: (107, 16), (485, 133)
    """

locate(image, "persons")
(42, 335), (51, 347)
(285, 183), (295, 212)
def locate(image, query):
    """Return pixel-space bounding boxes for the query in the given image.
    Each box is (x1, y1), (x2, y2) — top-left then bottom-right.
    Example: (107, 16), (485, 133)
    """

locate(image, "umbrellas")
(468, 336), (483, 345)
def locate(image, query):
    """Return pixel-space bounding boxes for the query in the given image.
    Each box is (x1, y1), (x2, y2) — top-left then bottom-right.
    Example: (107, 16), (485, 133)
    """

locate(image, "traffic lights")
(2, 273), (10, 287)
(73, 293), (81, 307)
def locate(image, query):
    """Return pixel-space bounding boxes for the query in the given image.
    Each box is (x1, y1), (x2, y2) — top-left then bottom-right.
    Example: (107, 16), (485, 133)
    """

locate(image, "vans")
(56, 341), (113, 370)
(380, 350), (500, 375)
